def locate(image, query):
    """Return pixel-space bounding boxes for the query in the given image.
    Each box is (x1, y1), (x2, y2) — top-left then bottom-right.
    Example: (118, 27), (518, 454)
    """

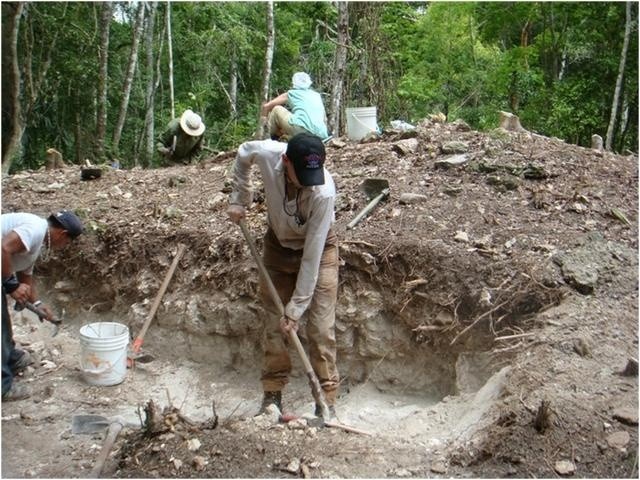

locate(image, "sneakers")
(315, 405), (339, 423)
(254, 389), (282, 417)
(1, 381), (34, 402)
(9, 348), (40, 370)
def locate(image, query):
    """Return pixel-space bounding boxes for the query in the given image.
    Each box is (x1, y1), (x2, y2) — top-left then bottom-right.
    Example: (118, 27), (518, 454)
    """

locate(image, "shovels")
(82, 158), (102, 182)
(239, 217), (342, 430)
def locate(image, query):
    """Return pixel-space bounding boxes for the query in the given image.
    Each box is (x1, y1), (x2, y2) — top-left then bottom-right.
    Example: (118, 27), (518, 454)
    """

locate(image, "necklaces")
(39, 226), (51, 262)
(280, 179), (302, 215)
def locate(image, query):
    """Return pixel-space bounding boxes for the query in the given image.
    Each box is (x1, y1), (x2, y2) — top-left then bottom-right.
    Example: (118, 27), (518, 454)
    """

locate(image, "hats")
(285, 132), (326, 188)
(49, 206), (84, 240)
(292, 71), (312, 89)
(179, 109), (206, 137)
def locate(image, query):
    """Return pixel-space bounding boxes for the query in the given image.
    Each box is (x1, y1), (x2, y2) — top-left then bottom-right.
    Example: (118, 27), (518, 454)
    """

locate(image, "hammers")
(169, 135), (177, 151)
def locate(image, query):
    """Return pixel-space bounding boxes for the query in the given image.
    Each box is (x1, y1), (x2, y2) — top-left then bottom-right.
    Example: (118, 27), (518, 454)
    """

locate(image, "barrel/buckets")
(74, 323), (130, 388)
(345, 106), (378, 140)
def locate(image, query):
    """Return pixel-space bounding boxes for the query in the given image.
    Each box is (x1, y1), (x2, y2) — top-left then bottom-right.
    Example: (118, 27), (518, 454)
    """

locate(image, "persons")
(222, 131), (344, 423)
(262, 70), (330, 143)
(155, 109), (206, 165)
(2, 210), (82, 399)
